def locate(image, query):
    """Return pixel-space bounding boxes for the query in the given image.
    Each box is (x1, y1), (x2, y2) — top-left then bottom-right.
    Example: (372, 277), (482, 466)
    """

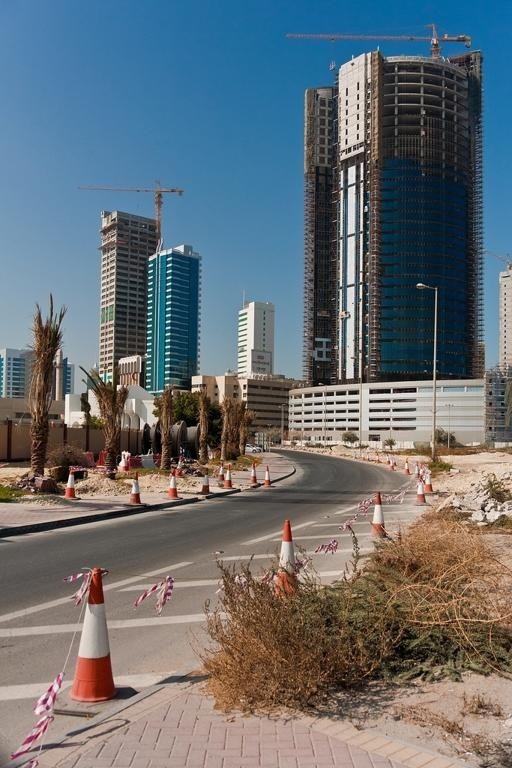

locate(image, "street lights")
(415, 282), (438, 461)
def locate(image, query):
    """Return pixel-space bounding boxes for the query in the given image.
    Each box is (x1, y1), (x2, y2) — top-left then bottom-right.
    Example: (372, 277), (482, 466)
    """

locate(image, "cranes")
(78, 181), (181, 238)
(286, 25), (471, 58)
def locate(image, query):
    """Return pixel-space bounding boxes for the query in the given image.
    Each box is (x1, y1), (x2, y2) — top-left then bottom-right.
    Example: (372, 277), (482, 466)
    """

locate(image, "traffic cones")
(64, 470), (82, 501)
(353, 451), (433, 506)
(272, 519), (301, 600)
(123, 471), (146, 507)
(371, 491), (386, 541)
(199, 468), (215, 495)
(163, 456), (183, 500)
(69, 568), (116, 701)
(219, 461), (233, 489)
(250, 455), (270, 487)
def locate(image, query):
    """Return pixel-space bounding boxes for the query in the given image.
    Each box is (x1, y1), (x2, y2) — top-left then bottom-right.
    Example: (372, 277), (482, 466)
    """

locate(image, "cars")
(245, 443), (263, 453)
